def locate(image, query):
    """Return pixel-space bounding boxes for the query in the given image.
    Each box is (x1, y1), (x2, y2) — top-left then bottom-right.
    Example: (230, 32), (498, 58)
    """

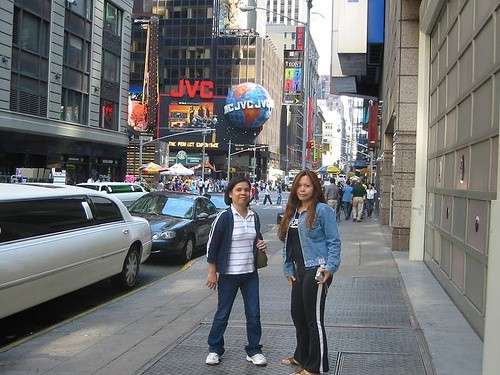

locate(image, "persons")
(134, 177), (228, 196)
(205, 176), (267, 367)
(251, 180), (288, 205)
(85, 174), (112, 183)
(278, 171), (341, 375)
(324, 176), (377, 223)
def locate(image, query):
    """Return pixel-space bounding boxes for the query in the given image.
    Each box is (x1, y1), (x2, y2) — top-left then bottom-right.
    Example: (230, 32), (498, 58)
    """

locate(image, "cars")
(126, 190), (224, 264)
(75, 181), (150, 211)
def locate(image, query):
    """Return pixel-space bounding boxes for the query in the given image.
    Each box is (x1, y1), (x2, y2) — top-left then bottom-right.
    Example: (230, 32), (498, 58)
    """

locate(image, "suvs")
(0, 179), (154, 334)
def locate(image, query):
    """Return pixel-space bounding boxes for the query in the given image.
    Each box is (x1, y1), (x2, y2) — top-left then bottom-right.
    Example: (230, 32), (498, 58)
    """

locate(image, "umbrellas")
(138, 162), (170, 174)
(314, 165), (341, 175)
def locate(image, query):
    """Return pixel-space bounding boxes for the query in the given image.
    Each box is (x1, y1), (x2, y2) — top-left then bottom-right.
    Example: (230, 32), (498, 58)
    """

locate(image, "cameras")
(314, 267), (324, 282)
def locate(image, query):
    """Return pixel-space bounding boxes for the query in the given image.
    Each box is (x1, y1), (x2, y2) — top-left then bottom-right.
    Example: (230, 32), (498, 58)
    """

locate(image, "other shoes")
(367, 217), (369, 218)
(353, 218), (357, 222)
(357, 220), (363, 222)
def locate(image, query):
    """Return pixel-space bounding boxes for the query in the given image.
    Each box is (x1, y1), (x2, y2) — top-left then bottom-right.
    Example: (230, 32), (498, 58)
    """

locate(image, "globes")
(224, 81), (273, 130)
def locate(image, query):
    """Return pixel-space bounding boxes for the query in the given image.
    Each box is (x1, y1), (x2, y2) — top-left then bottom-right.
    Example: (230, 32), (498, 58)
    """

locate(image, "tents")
(160, 163), (195, 178)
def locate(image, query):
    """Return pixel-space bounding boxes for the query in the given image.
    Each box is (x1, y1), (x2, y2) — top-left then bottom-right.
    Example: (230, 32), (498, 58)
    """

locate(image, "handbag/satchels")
(254, 210), (269, 268)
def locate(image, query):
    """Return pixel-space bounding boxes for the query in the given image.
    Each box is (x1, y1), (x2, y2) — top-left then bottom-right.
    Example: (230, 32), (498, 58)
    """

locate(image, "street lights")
(240, 1), (314, 170)
(192, 115), (218, 196)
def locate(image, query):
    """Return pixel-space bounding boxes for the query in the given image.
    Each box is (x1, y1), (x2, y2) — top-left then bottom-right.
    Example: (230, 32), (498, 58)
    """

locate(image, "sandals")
(281, 357), (302, 365)
(289, 368), (321, 375)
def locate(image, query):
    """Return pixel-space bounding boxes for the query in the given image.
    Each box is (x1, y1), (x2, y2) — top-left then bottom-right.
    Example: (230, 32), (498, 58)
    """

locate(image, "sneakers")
(246, 353), (267, 365)
(206, 352), (220, 364)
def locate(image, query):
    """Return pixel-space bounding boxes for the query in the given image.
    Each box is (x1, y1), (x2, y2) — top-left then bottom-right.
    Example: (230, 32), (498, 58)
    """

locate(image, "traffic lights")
(310, 139), (314, 149)
